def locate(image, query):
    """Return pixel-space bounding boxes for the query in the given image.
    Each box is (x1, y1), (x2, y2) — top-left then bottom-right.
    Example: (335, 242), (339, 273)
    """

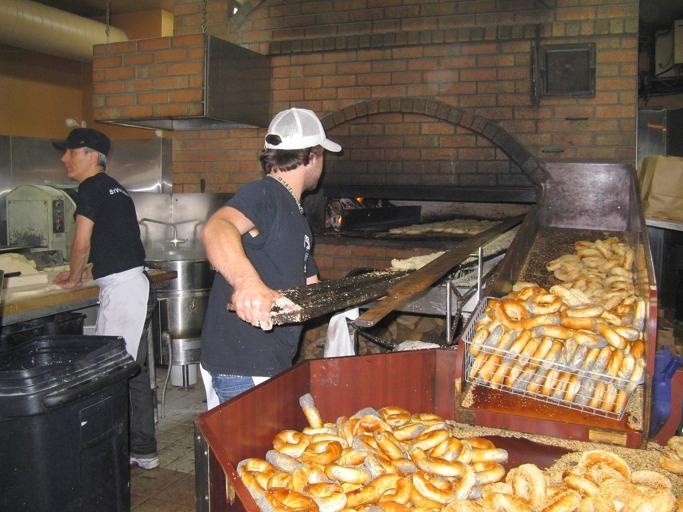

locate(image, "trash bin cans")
(0, 311), (141, 512)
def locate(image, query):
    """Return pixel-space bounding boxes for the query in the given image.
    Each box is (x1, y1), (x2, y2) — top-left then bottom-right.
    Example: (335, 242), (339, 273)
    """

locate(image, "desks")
(1, 268), (179, 428)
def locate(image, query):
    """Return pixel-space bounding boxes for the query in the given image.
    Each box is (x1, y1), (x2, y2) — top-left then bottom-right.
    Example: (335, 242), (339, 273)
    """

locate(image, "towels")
(323, 305), (360, 358)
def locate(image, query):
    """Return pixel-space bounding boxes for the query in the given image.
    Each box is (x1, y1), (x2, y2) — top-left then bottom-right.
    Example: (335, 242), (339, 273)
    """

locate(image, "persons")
(198, 105), (344, 407)
(44, 124), (162, 472)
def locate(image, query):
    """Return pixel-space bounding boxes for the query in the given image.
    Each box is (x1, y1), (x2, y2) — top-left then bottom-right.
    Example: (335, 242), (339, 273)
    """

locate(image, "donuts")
(470, 236), (645, 414)
(236, 406), (683, 512)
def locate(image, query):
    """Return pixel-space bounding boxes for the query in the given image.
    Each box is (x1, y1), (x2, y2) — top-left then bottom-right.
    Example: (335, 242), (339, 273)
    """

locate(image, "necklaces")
(272, 172), (305, 215)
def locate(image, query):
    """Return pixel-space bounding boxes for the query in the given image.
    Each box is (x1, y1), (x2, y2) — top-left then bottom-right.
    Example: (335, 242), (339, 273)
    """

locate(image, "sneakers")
(130, 455), (160, 470)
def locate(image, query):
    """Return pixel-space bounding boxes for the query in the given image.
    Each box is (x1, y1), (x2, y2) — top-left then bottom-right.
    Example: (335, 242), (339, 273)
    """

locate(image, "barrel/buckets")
(0, 334), (145, 511)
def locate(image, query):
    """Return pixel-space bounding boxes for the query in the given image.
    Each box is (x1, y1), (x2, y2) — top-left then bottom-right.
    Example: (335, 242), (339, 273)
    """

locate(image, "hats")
(52, 127), (112, 156)
(264, 106), (343, 153)
